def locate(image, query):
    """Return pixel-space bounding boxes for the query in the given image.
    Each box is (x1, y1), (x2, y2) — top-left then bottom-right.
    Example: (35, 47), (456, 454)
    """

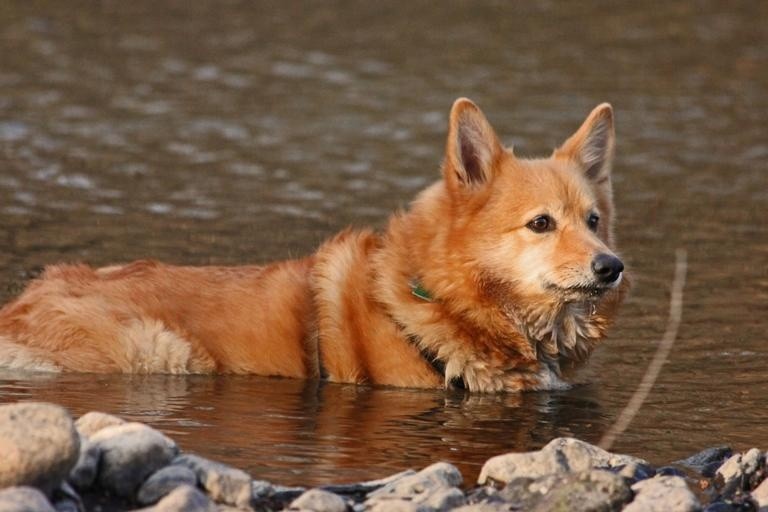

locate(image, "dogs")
(1, 96), (634, 396)
